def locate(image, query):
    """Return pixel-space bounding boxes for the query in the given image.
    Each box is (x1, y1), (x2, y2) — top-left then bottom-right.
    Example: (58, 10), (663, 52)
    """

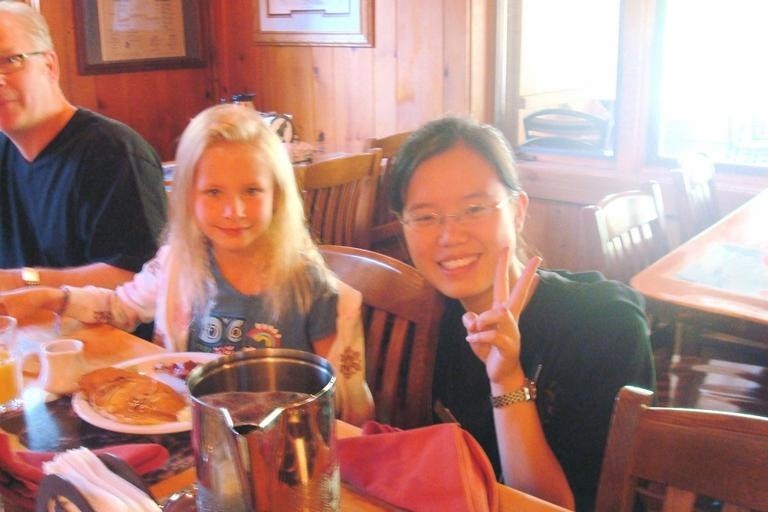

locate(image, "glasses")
(0, 47), (50, 75)
(387, 192), (514, 234)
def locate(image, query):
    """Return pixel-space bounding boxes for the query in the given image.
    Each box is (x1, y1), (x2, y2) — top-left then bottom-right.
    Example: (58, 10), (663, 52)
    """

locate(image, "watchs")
(21, 262), (43, 288)
(53, 285), (70, 318)
(490, 377), (541, 409)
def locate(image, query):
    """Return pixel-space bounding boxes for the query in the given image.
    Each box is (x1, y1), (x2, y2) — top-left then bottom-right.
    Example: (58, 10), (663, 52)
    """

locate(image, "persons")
(376, 110), (658, 512)
(0, 2), (169, 343)
(0, 104), (374, 426)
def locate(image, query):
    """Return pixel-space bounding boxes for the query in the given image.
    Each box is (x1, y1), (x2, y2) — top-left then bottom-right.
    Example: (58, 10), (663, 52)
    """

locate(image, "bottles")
(234, 92), (259, 111)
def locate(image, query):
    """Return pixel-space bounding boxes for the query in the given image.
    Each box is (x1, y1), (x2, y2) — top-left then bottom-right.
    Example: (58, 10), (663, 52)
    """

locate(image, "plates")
(70, 350), (221, 435)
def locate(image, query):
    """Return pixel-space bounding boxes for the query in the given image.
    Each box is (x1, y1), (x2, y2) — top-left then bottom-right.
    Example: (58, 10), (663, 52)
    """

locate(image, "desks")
(0, 312), (572, 512)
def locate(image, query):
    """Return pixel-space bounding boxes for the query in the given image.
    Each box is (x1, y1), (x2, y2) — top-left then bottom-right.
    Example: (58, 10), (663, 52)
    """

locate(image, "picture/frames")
(252, 0), (375, 48)
(71, 0), (206, 75)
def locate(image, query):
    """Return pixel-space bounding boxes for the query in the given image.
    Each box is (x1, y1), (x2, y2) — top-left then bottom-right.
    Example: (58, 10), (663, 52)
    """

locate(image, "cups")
(40, 338), (87, 395)
(0, 315), (27, 413)
(21, 309), (64, 382)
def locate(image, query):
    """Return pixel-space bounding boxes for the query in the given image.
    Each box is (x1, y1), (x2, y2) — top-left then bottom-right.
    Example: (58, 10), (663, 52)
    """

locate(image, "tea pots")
(185, 346), (337, 512)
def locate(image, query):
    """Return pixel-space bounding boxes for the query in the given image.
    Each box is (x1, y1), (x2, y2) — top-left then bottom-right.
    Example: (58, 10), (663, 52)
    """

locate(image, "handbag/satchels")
(335, 420), (499, 511)
